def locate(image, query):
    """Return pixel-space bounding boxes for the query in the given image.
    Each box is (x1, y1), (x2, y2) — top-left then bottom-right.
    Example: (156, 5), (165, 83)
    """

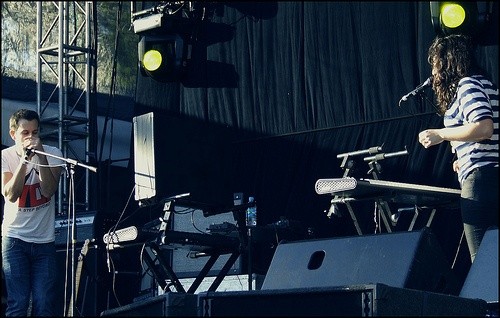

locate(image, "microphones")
(25, 148), (32, 160)
(402, 75), (434, 100)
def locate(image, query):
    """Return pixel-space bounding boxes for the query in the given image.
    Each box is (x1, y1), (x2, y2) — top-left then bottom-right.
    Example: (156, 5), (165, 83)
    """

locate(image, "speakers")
(53, 245), (115, 318)
(259, 230), (500, 309)
(169, 206), (243, 278)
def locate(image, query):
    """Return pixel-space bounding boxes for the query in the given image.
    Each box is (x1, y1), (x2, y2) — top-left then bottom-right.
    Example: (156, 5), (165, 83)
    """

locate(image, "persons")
(419, 35), (500, 263)
(1, 109), (63, 317)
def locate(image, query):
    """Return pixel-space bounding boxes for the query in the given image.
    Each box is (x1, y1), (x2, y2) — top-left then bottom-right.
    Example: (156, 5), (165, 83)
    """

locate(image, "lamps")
(429, 1), (478, 39)
(132, 7), (181, 78)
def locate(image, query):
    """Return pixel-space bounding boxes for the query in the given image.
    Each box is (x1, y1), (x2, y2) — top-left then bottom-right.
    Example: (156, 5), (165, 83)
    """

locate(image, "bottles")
(245, 197), (257, 226)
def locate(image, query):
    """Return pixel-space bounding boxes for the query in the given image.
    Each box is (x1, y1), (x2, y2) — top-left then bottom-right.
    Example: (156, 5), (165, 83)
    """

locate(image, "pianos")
(316, 175), (470, 286)
(104, 228), (248, 300)
